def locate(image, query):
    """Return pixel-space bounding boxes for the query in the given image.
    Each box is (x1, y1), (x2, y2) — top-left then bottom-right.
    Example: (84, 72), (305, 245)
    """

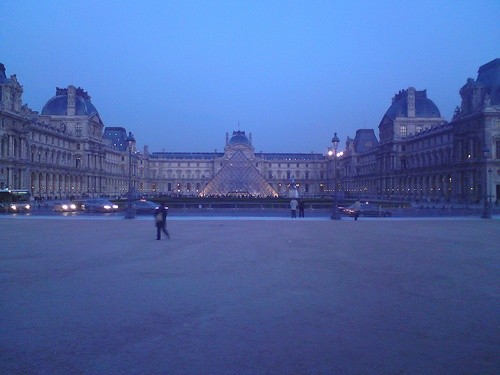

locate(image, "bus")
(0, 189), (34, 213)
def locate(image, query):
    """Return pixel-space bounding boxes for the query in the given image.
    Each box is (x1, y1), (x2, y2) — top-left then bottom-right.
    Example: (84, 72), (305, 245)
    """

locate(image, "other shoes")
(156, 238), (161, 240)
(167, 233), (170, 239)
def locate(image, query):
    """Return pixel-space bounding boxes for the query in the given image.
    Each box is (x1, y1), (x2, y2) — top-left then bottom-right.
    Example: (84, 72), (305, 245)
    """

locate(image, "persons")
(298, 196), (304, 218)
(12, 75), (23, 112)
(154, 201), (170, 240)
(290, 197), (298, 218)
(354, 197), (361, 220)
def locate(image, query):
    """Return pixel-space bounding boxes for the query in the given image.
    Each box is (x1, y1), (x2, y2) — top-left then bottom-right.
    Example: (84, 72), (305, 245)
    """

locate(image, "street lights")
(331, 132), (342, 219)
(125, 131), (136, 218)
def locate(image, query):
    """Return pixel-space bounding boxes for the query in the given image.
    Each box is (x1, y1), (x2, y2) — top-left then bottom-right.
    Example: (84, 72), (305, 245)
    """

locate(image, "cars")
(84, 198), (119, 212)
(342, 204), (392, 218)
(53, 200), (77, 212)
(133, 200), (169, 215)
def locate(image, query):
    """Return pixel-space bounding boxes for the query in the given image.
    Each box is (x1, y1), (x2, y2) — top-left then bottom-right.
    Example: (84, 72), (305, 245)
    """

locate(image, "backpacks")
(156, 212), (164, 222)
